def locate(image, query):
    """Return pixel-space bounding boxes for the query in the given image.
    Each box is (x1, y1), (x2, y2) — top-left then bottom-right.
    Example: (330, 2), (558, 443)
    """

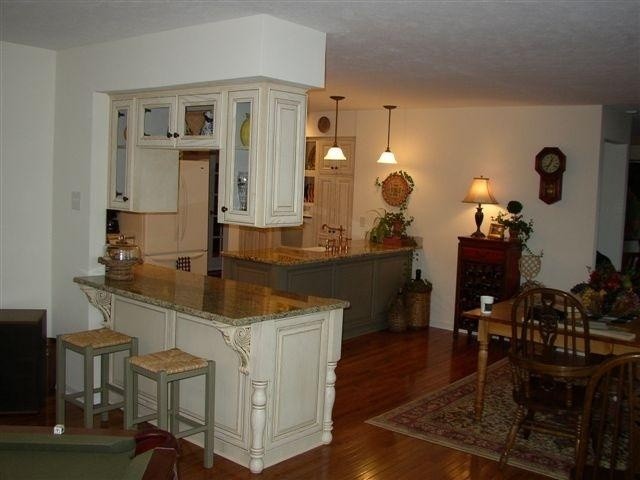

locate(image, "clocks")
(535, 146), (567, 204)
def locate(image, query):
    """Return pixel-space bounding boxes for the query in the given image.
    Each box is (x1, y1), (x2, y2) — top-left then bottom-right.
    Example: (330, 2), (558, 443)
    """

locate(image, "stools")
(54, 327), (139, 432)
(123, 347), (216, 470)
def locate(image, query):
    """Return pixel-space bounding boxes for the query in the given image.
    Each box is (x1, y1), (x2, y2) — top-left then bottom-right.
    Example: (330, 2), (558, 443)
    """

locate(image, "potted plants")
(363, 206), (415, 248)
(506, 199), (524, 241)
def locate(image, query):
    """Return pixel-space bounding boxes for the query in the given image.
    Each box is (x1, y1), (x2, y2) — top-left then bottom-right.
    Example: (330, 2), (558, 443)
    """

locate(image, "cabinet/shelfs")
(218, 80), (309, 230)
(315, 136), (356, 177)
(134, 87), (221, 152)
(314, 173), (354, 248)
(237, 226), (273, 251)
(305, 137), (316, 208)
(104, 95), (181, 213)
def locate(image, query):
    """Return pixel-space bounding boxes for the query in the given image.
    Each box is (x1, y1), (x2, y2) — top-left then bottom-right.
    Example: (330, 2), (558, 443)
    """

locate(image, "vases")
(581, 289), (600, 315)
(236, 170), (249, 210)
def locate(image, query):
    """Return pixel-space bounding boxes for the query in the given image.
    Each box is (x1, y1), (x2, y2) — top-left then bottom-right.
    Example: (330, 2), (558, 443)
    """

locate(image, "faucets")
(321, 224), (344, 255)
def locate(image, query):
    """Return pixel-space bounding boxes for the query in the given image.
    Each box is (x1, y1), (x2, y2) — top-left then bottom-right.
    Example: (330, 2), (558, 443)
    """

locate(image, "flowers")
(570, 250), (635, 317)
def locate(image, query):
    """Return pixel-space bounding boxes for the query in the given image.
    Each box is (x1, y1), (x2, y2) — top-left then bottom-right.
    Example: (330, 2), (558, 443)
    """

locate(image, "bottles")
(238, 175), (247, 211)
(107, 212), (120, 233)
(240, 113), (250, 145)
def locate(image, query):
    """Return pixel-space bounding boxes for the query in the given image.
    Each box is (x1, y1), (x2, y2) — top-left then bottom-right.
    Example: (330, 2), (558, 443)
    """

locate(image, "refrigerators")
(117, 159), (209, 277)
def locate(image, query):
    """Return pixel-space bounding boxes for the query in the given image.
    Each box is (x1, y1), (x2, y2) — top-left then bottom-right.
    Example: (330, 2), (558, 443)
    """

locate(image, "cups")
(480, 295), (494, 314)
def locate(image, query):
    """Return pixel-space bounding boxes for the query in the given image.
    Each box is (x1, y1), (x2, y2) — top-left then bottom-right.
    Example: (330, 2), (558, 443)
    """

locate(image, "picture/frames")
(485, 221), (506, 240)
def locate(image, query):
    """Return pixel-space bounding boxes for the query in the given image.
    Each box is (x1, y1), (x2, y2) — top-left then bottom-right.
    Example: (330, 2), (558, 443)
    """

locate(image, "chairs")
(575, 354), (640, 479)
(496, 287), (598, 471)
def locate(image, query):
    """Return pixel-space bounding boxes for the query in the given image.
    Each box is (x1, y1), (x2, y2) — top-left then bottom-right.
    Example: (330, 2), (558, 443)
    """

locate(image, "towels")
(175, 256), (191, 273)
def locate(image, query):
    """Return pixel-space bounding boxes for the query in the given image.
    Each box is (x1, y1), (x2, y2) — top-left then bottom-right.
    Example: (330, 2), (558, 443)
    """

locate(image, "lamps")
(376, 104), (398, 165)
(462, 174), (498, 237)
(323, 96), (349, 161)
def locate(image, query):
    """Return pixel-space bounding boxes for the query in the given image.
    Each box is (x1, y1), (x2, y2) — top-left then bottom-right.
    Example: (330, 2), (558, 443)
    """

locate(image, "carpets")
(363, 352), (640, 480)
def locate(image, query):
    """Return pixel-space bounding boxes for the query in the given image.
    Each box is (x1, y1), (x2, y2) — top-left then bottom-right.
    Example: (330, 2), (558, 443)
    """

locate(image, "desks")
(0, 423), (180, 480)
(0, 307), (56, 425)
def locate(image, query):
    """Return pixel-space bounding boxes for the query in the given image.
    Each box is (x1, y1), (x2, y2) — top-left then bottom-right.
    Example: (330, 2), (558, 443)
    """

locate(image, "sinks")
(301, 246), (338, 252)
